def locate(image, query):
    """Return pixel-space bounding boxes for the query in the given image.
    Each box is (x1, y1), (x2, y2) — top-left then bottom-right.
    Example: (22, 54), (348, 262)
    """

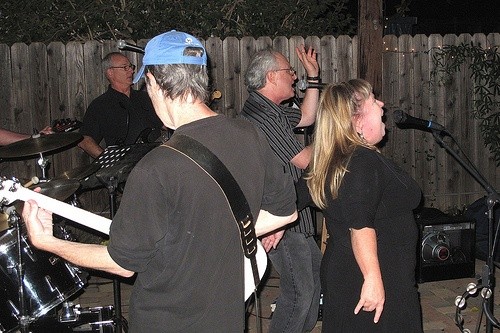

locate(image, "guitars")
(0, 176), (269, 304)
(118, 91), (222, 194)
(50, 119), (82, 135)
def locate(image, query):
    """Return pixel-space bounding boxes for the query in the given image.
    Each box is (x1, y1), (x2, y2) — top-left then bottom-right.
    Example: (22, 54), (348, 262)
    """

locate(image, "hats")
(131, 30), (207, 84)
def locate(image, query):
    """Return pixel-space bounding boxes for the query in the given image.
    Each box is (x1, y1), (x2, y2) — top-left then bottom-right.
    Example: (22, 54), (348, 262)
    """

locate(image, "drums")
(0, 218), (88, 333)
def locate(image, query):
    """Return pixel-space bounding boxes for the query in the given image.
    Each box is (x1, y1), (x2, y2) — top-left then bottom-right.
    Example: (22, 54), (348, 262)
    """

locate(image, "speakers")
(414, 216), (477, 283)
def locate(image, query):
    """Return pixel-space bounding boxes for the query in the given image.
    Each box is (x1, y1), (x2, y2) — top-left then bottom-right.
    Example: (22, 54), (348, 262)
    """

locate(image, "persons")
(20, 29), (300, 332)
(1, 127), (54, 145)
(261, 78), (423, 333)
(77, 52), (163, 159)
(239, 44), (323, 333)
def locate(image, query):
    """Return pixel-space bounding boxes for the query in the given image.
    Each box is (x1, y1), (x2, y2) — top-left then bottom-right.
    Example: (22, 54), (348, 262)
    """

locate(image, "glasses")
(272, 67), (297, 77)
(109, 64), (136, 72)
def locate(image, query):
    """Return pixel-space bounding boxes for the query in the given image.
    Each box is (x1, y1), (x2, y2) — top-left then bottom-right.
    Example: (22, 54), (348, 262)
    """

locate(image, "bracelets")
(307, 75), (320, 81)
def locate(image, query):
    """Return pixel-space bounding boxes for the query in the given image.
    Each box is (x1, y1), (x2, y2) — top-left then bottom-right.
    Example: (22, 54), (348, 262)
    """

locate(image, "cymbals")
(452, 283), (492, 333)
(0, 133), (84, 162)
(0, 180), (81, 233)
(51, 164), (103, 182)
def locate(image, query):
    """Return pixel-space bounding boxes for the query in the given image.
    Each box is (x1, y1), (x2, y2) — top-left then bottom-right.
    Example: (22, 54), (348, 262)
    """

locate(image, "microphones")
(393, 110), (447, 132)
(298, 80), (327, 90)
(118, 40), (145, 55)
